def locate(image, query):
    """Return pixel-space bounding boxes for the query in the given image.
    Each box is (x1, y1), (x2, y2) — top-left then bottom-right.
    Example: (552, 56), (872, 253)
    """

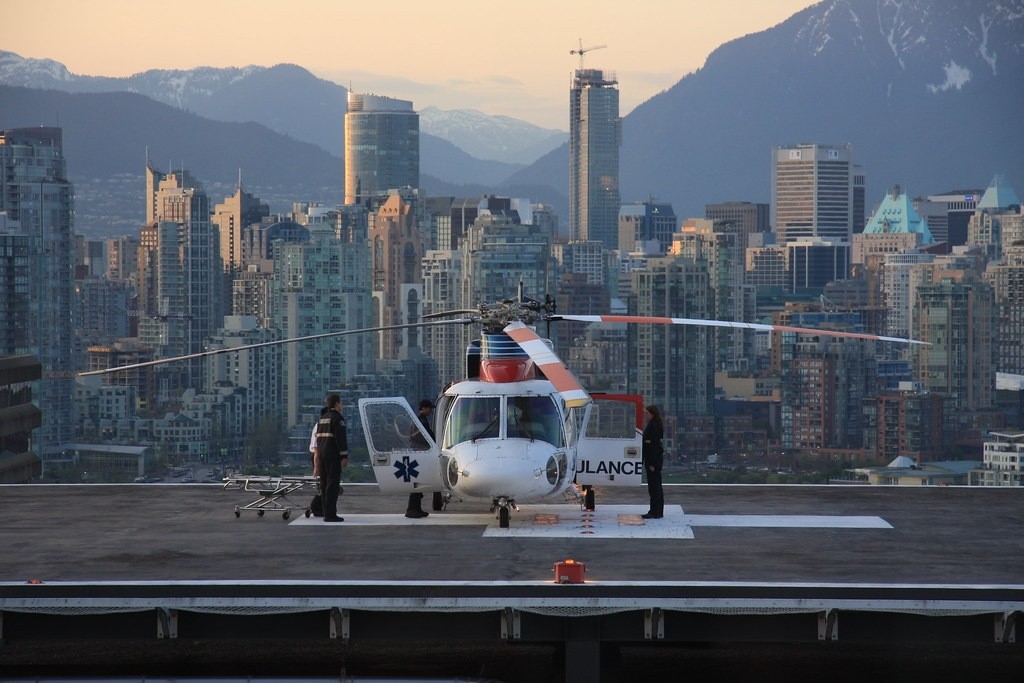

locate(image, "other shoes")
(641, 512), (663, 518)
(327, 515), (343, 522)
(405, 508), (429, 518)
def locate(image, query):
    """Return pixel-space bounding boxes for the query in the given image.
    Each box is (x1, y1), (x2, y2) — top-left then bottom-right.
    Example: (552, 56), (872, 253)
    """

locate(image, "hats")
(419, 399), (436, 408)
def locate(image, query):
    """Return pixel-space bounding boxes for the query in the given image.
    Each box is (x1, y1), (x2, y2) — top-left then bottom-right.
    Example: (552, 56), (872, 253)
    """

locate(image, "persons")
(641, 406), (664, 519)
(317, 394), (348, 522)
(309, 407), (328, 518)
(405, 400), (437, 518)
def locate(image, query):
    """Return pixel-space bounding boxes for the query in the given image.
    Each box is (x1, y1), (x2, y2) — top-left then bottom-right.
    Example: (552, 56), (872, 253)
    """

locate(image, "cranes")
(570, 37), (607, 69)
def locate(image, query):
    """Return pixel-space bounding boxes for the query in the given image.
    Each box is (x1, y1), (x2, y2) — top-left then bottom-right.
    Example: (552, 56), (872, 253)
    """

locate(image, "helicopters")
(78, 281), (932, 527)
